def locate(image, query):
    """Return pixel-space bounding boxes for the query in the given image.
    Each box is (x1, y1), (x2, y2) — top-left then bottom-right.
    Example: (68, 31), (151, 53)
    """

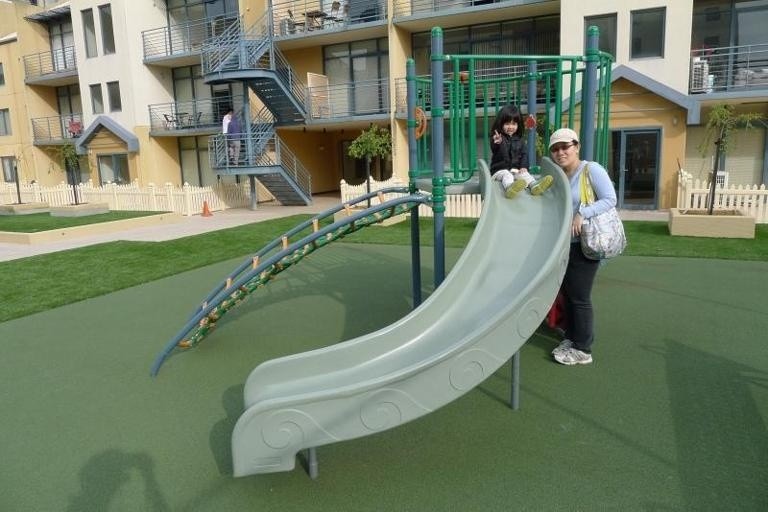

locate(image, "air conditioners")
(709, 171), (730, 208)
(691, 61), (709, 93)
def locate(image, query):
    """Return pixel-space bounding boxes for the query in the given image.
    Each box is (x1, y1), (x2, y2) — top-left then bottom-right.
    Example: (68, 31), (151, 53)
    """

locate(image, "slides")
(231, 157), (574, 477)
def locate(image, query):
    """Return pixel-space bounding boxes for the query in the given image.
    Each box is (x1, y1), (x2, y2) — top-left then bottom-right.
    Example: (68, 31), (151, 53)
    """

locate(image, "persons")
(547, 128), (620, 365)
(490, 104), (554, 201)
(222, 110), (234, 143)
(226, 116), (241, 164)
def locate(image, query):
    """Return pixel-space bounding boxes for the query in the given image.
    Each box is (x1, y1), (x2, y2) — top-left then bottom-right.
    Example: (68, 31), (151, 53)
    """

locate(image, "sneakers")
(504, 179), (526, 199)
(530, 175), (553, 194)
(551, 339), (593, 365)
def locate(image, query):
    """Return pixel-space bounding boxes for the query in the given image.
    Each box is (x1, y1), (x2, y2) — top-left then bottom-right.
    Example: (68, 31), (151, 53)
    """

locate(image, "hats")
(548, 128), (579, 149)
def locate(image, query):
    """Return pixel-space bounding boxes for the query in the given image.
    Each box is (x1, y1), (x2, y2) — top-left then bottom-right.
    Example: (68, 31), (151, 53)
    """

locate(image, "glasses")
(552, 144), (573, 153)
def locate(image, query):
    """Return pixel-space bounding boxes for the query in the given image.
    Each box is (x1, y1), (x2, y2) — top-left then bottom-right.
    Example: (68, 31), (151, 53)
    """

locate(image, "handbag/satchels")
(579, 202), (627, 261)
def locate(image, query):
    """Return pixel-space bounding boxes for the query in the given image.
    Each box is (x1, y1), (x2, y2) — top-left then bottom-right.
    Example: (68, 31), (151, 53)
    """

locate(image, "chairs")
(163, 111), (202, 130)
(287, 8), (345, 33)
